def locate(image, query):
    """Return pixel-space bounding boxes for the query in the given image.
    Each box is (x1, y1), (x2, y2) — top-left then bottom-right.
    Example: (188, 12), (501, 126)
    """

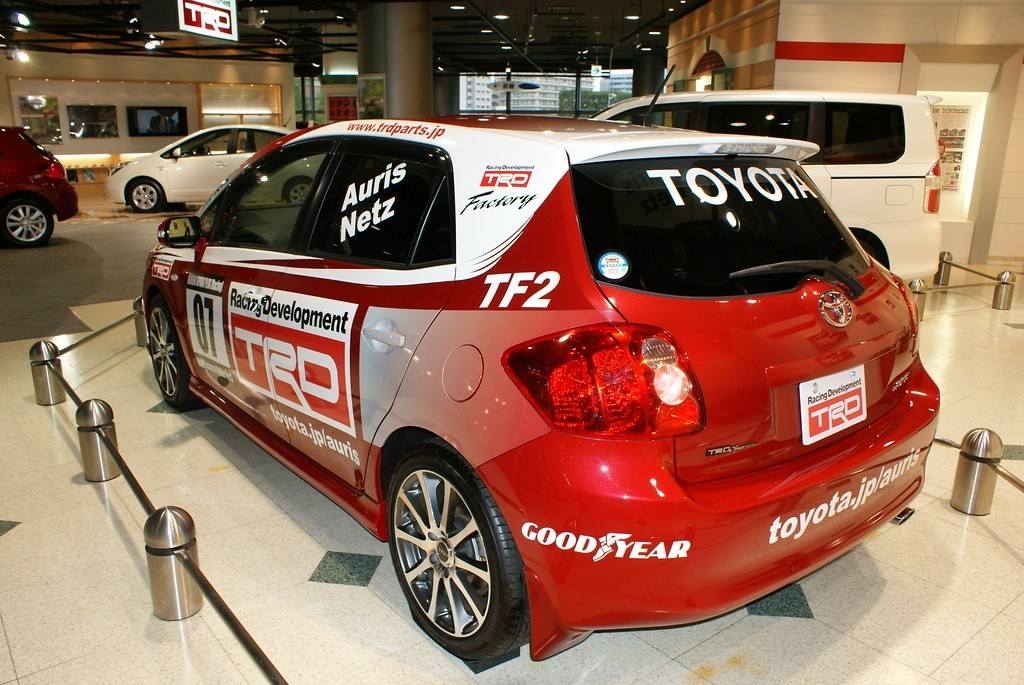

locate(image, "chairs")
(239, 138), (246, 153)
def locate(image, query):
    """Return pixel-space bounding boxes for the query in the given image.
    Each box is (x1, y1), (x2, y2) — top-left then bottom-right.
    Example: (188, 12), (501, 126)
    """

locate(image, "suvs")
(0, 124), (78, 249)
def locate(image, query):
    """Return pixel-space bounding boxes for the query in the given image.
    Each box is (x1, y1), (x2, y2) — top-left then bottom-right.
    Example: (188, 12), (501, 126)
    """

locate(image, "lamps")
(138, 31), (161, 50)
(4, 51), (16, 61)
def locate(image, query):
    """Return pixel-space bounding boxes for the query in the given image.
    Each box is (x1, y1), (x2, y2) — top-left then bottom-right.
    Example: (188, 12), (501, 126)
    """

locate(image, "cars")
(104, 123), (327, 214)
(139, 116), (940, 666)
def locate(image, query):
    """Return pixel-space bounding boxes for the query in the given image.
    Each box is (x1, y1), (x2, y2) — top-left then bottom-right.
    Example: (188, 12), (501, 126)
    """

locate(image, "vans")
(584, 90), (941, 284)
